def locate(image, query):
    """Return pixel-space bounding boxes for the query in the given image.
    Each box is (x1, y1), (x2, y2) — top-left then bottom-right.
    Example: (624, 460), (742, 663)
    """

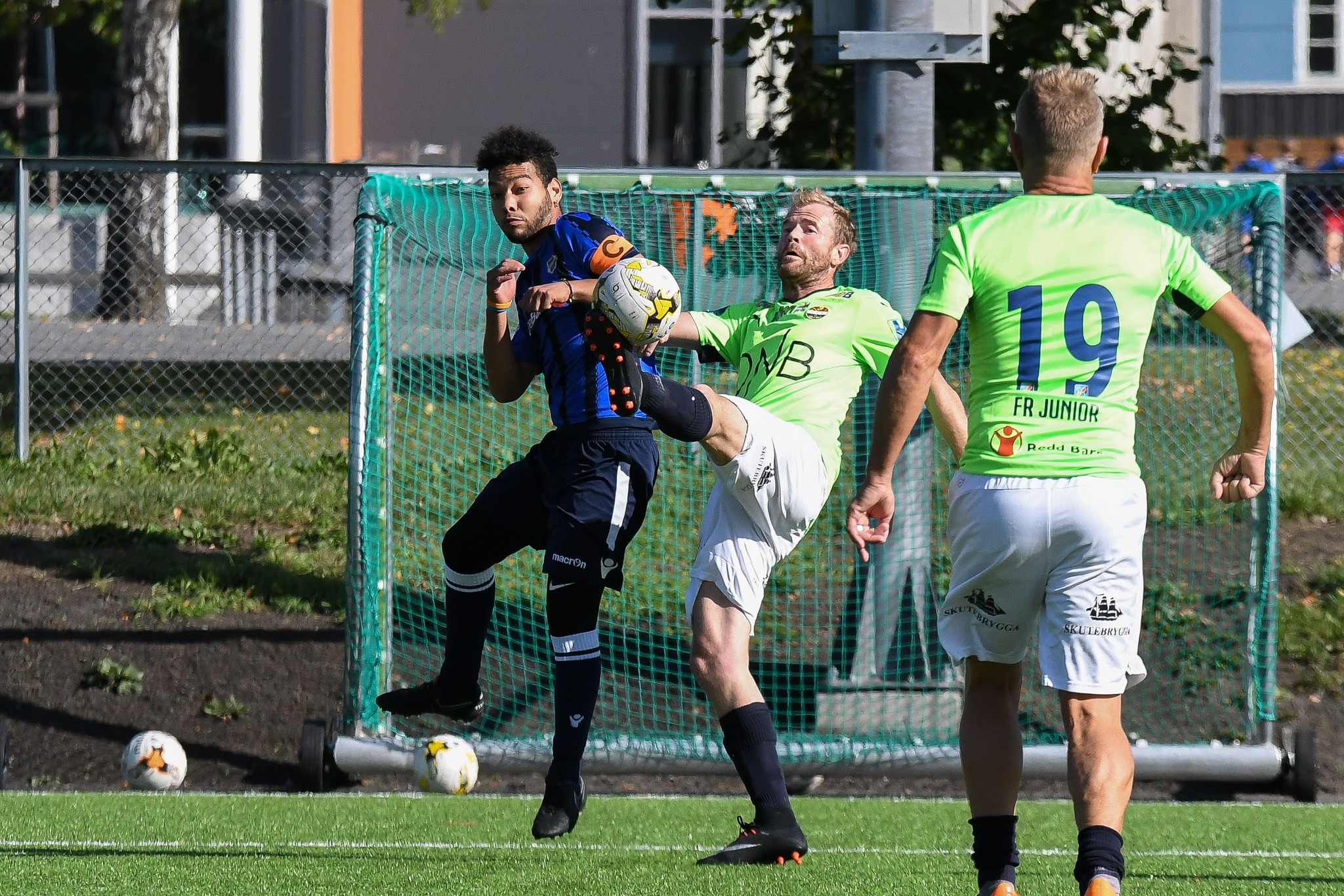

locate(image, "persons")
(377, 122), (662, 840)
(575, 187), (969, 866)
(1233, 136), (1344, 281)
(842, 61), (1276, 896)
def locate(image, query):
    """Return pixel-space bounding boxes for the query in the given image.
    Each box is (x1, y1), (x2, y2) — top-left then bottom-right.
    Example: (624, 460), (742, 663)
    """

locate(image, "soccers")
(593, 258), (683, 346)
(414, 733), (479, 794)
(122, 731), (189, 792)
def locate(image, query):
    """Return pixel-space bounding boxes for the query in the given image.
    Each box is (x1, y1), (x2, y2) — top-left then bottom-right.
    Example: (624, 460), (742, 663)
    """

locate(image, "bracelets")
(486, 298), (513, 313)
(558, 278), (574, 304)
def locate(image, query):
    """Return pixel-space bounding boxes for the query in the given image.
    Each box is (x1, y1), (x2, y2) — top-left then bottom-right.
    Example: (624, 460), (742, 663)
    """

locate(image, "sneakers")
(376, 674), (485, 723)
(580, 309), (643, 418)
(531, 776), (587, 840)
(695, 816), (808, 865)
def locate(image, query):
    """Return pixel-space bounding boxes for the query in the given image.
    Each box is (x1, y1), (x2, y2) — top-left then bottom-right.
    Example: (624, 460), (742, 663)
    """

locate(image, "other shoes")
(1079, 867), (1121, 896)
(977, 879), (1018, 896)
